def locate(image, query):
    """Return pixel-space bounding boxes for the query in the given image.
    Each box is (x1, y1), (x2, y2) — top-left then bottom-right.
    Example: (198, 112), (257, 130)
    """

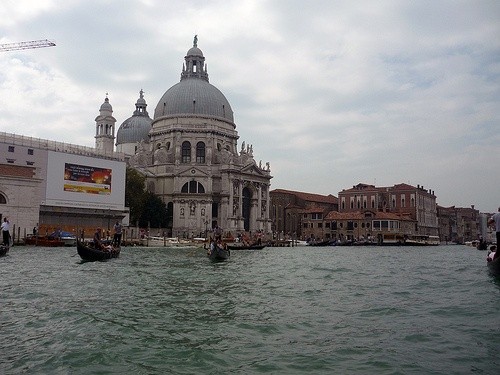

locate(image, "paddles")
(186, 231), (223, 256)
(70, 230), (122, 257)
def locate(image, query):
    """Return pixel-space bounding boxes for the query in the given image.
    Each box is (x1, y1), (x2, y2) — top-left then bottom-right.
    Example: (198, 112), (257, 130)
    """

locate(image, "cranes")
(0, 40), (56, 53)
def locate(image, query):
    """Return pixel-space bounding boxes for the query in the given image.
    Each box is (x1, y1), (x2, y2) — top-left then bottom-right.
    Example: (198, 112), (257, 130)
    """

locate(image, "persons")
(33, 227), (36, 235)
(86, 220), (123, 253)
(237, 230), (264, 245)
(183, 232), (186, 238)
(157, 232), (161, 240)
(141, 229), (150, 239)
(487, 208), (500, 260)
(50, 228), (57, 238)
(213, 226), (223, 244)
(0, 217), (10, 246)
(210, 239), (215, 251)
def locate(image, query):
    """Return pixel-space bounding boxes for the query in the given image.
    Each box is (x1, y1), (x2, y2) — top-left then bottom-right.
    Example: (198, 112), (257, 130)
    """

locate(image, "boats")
(76, 238), (121, 260)
(208, 243), (231, 262)
(227, 244), (267, 250)
